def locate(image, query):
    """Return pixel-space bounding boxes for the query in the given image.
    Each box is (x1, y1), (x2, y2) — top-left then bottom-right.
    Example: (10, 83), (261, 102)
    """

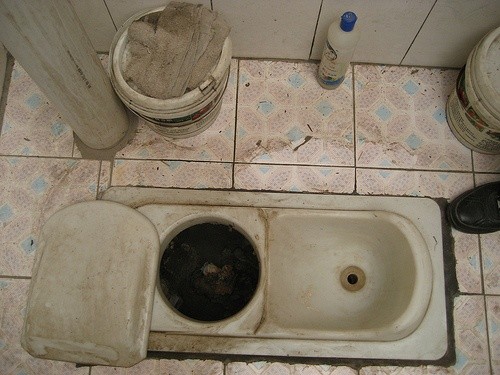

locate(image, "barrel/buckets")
(445, 26), (500, 154)
(108, 4), (231, 139)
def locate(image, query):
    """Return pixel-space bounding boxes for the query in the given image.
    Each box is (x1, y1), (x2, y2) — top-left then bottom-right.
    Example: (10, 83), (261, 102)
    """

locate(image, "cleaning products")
(316, 12), (361, 91)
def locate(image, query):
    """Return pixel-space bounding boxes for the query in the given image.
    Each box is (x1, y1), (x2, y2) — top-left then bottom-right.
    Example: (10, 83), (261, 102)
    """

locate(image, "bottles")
(316, 12), (359, 91)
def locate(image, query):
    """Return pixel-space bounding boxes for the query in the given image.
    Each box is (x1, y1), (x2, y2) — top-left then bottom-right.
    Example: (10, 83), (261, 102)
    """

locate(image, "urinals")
(99, 191), (454, 357)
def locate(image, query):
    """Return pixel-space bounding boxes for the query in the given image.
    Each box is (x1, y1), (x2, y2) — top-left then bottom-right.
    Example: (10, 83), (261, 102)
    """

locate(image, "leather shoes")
(447, 181), (500, 234)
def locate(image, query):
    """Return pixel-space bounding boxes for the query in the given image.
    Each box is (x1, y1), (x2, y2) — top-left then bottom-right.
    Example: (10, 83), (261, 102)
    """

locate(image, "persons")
(445, 177), (500, 234)
